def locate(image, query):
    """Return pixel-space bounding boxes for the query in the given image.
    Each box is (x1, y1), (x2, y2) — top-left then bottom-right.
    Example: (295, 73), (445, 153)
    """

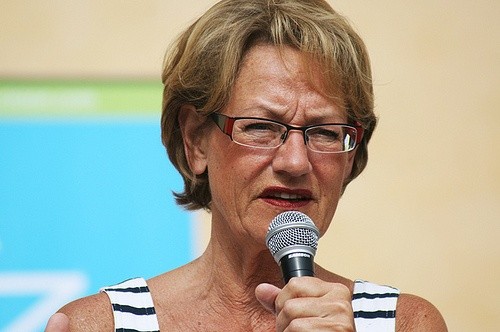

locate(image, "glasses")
(210, 112), (365, 154)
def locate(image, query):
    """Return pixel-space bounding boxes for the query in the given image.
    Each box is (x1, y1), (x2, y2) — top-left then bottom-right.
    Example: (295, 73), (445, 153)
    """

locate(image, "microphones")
(265, 211), (320, 286)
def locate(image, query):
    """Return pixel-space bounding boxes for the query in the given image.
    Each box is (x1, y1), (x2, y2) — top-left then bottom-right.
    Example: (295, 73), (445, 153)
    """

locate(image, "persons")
(43, 0), (448, 332)
